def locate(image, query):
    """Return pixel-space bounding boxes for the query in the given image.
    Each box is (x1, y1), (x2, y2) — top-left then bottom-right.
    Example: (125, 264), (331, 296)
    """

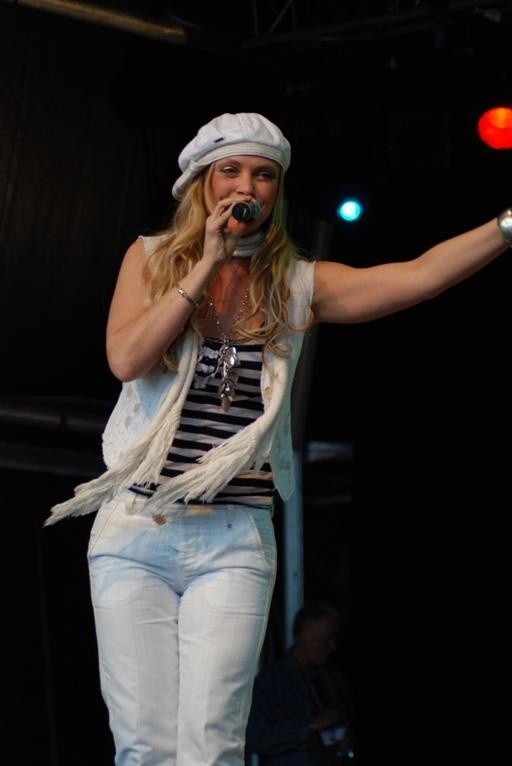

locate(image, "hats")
(171, 111), (291, 202)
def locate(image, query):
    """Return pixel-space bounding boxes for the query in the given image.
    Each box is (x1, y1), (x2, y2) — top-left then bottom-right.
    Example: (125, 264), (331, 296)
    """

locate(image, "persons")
(42, 110), (512, 765)
(246, 599), (361, 765)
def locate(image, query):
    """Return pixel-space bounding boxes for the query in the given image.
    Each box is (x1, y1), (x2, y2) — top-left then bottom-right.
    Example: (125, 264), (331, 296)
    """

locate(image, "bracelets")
(497, 207), (512, 250)
(173, 280), (199, 308)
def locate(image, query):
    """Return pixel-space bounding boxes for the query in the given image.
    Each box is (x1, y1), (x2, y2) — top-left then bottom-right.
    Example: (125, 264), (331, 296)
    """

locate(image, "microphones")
(233, 195), (263, 227)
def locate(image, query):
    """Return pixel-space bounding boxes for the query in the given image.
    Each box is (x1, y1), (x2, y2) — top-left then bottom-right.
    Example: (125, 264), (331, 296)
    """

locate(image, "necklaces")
(205, 280), (249, 401)
